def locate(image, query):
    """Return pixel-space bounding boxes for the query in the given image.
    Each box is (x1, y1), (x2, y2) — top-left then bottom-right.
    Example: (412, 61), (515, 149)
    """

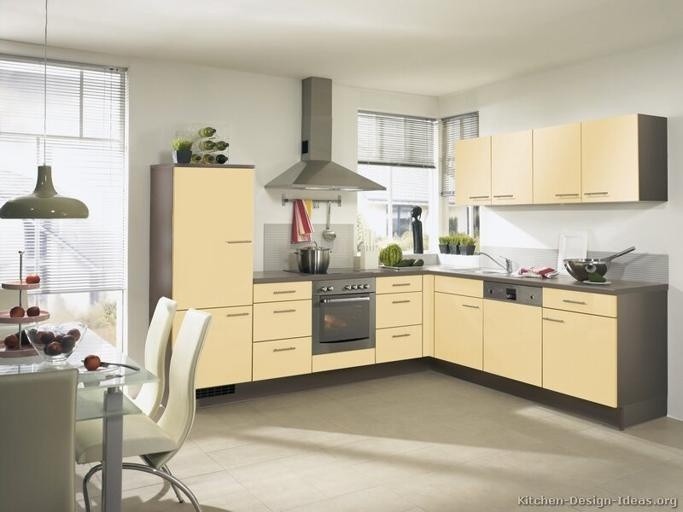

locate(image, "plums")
(3, 275), (80, 356)
(84, 355), (101, 371)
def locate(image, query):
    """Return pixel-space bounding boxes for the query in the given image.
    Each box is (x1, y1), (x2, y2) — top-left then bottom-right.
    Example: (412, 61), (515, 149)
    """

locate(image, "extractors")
(263, 76), (387, 193)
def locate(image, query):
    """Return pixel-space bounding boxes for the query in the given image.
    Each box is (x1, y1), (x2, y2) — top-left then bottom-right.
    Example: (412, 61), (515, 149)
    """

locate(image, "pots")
(294, 242), (332, 275)
(564, 246), (636, 283)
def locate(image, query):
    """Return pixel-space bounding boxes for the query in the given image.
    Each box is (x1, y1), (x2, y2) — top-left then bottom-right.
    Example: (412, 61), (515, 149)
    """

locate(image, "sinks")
(453, 268), (479, 273)
(482, 271), (498, 274)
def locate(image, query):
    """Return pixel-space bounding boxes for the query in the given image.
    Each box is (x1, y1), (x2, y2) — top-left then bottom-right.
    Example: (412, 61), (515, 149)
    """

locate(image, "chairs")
(74, 306), (212, 512)
(74, 295), (186, 512)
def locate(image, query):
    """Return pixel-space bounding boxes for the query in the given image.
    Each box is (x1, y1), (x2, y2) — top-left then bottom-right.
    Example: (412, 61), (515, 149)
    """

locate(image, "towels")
(514, 265), (559, 281)
(292, 199), (315, 244)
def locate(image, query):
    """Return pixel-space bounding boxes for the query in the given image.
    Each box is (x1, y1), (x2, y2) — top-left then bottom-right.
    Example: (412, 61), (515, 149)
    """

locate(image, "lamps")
(0, 0), (91, 220)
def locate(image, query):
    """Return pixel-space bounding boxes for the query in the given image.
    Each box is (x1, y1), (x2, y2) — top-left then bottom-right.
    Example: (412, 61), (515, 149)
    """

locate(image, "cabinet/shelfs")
(254, 281), (313, 381)
(150, 168), (254, 307)
(455, 129), (534, 205)
(148, 305), (254, 391)
(375, 275), (423, 364)
(543, 286), (667, 410)
(533, 113), (669, 205)
(434, 276), (484, 373)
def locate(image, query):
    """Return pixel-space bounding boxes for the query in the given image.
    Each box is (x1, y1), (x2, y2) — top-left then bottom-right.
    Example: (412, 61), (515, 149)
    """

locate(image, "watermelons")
(380, 244), (402, 266)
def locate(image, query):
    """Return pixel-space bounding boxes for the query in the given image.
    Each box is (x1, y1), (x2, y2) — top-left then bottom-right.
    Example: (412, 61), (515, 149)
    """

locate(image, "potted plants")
(171, 137), (194, 163)
(448, 237), (460, 255)
(459, 236), (475, 255)
(439, 236), (449, 254)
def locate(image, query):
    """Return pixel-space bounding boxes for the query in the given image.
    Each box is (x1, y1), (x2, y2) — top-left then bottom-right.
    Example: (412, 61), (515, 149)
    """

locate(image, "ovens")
(310, 277), (375, 356)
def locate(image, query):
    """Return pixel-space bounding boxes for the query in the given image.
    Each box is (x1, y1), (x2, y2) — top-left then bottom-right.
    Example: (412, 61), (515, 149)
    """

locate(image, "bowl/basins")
(23, 322), (86, 363)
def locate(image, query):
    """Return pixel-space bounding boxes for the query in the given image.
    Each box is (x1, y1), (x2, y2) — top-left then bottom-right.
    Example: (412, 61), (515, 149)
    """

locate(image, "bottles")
(190, 125), (230, 165)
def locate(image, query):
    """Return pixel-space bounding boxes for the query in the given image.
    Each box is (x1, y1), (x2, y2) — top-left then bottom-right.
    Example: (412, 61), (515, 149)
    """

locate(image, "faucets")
(475, 251), (512, 273)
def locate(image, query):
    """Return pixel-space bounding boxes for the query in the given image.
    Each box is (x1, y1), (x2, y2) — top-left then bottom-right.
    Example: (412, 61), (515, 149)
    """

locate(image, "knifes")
(81, 360), (137, 373)
